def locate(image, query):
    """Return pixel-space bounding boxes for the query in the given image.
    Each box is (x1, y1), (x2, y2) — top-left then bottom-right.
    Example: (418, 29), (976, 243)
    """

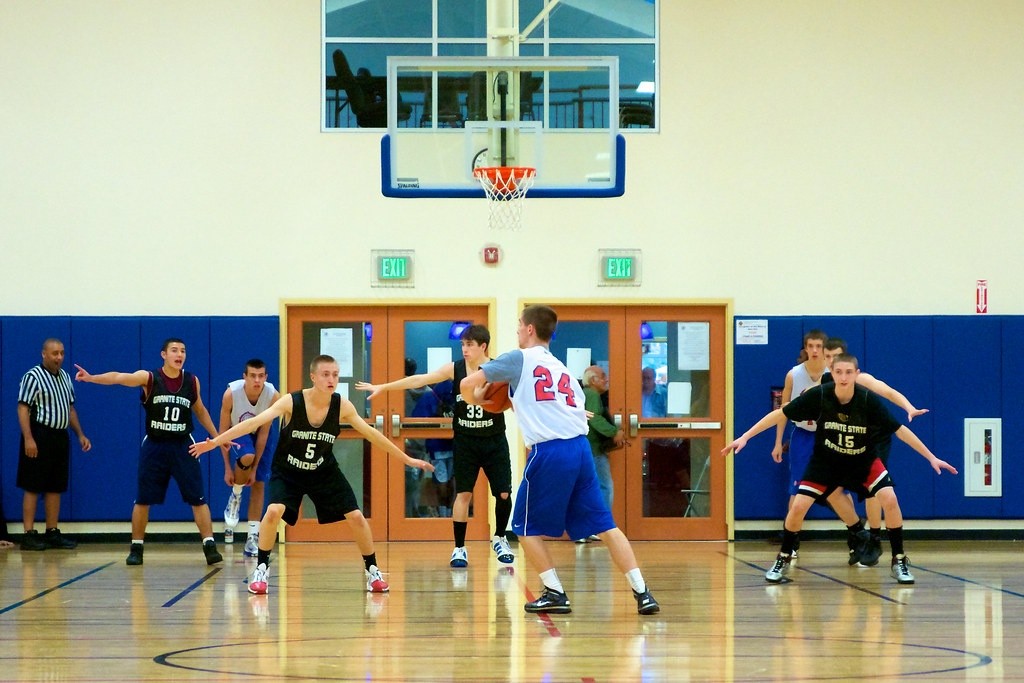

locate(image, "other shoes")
(589, 535), (601, 540)
(0, 538), (15, 549)
(575, 538), (587, 542)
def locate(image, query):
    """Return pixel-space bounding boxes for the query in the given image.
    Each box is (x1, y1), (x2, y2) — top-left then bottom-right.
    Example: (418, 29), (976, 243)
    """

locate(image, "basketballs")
(480, 381), (511, 414)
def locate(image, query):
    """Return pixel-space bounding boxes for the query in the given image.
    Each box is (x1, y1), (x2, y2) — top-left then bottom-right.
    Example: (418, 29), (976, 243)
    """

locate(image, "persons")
(403, 358), (472, 518)
(773, 328), (929, 565)
(219, 359), (279, 556)
(188, 355), (435, 594)
(460, 305), (660, 614)
(0, 494), (15, 549)
(579, 360), (687, 517)
(720, 352), (959, 583)
(15, 337), (92, 551)
(73, 338), (231, 564)
(354, 325), (514, 566)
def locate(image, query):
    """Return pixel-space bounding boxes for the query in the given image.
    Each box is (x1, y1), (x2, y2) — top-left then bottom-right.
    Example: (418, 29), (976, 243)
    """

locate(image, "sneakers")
(494, 567), (514, 585)
(860, 542), (881, 565)
(848, 529), (869, 565)
(248, 593), (270, 616)
(525, 585), (571, 613)
(890, 554), (914, 583)
(492, 535), (515, 565)
(126, 543), (144, 565)
(365, 564), (390, 592)
(224, 490), (243, 527)
(203, 540), (223, 565)
(248, 563), (270, 594)
(632, 585), (659, 614)
(365, 592), (389, 617)
(20, 530), (46, 550)
(765, 554), (792, 583)
(244, 534), (258, 557)
(44, 529), (76, 549)
(450, 546), (468, 567)
(791, 550), (799, 558)
(450, 571), (467, 588)
